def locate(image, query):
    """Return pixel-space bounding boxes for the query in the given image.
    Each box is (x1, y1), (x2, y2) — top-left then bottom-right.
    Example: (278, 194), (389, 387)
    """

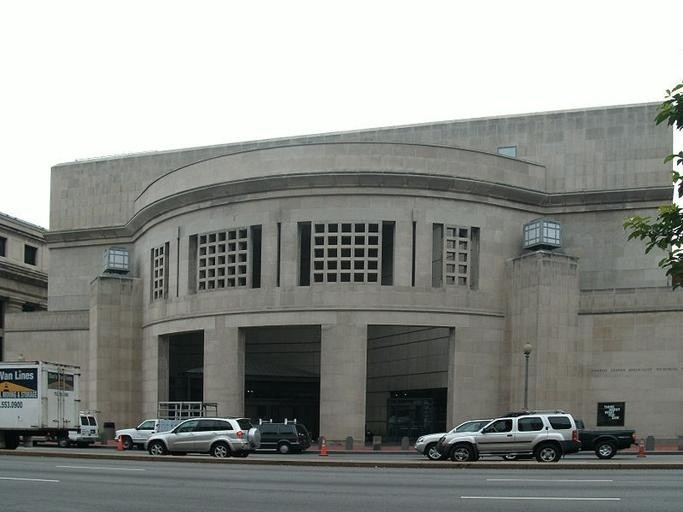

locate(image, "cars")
(253, 423), (311, 455)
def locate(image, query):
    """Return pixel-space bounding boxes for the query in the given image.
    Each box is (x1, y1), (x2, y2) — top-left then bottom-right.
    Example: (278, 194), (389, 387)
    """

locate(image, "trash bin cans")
(373, 436), (381, 450)
(103, 421), (114, 445)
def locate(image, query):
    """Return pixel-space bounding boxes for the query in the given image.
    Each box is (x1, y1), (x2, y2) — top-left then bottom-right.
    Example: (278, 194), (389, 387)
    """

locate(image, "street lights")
(522, 341), (532, 410)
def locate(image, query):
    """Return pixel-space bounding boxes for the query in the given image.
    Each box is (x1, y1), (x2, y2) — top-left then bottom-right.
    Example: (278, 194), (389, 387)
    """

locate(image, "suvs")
(144, 417), (261, 458)
(436, 410), (581, 462)
(415, 417), (493, 462)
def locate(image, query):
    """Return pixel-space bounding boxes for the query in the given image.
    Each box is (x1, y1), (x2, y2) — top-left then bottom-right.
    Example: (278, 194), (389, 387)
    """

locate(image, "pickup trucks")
(576, 420), (635, 461)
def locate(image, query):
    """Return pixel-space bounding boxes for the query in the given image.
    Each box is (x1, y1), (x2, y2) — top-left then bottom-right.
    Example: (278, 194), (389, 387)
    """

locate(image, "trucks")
(19, 410), (99, 448)
(115, 401), (217, 449)
(0, 360), (79, 449)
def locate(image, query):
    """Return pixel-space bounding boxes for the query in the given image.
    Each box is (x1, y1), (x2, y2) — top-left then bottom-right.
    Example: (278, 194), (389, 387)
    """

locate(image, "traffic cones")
(319, 439), (329, 457)
(639, 440), (647, 456)
(116, 437), (123, 450)
